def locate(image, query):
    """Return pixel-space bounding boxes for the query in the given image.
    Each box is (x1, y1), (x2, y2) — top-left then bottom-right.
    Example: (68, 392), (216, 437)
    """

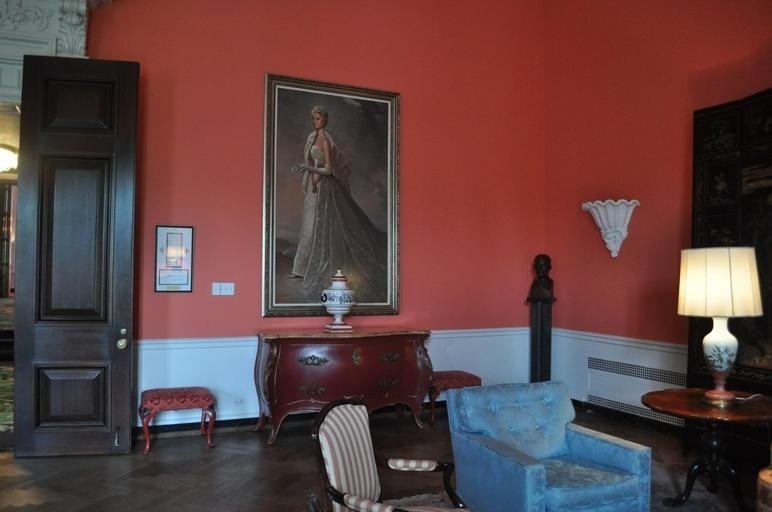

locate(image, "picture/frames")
(154, 224), (193, 291)
(260, 72), (401, 317)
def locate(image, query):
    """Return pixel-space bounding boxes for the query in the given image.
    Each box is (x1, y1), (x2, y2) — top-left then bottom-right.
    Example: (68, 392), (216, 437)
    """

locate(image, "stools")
(757, 465), (772, 512)
(138, 387), (216, 456)
(427, 371), (481, 423)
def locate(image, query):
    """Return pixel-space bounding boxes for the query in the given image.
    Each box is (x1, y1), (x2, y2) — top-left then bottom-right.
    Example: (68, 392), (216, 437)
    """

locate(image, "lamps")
(677, 247), (763, 400)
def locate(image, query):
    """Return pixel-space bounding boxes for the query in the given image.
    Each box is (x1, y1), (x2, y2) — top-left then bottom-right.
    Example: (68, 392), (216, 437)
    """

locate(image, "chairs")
(305, 397), (469, 512)
(445, 379), (652, 512)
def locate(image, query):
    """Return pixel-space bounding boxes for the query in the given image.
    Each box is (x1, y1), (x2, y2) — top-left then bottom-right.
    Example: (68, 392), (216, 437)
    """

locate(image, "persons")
(291, 106), (386, 300)
(530, 255), (553, 296)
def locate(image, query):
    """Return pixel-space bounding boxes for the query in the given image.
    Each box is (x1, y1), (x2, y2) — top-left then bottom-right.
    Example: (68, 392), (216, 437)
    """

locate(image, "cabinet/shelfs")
(254, 328), (433, 445)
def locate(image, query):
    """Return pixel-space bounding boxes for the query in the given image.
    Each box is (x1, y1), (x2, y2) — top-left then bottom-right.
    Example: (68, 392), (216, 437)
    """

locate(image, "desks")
(641, 387), (772, 512)
